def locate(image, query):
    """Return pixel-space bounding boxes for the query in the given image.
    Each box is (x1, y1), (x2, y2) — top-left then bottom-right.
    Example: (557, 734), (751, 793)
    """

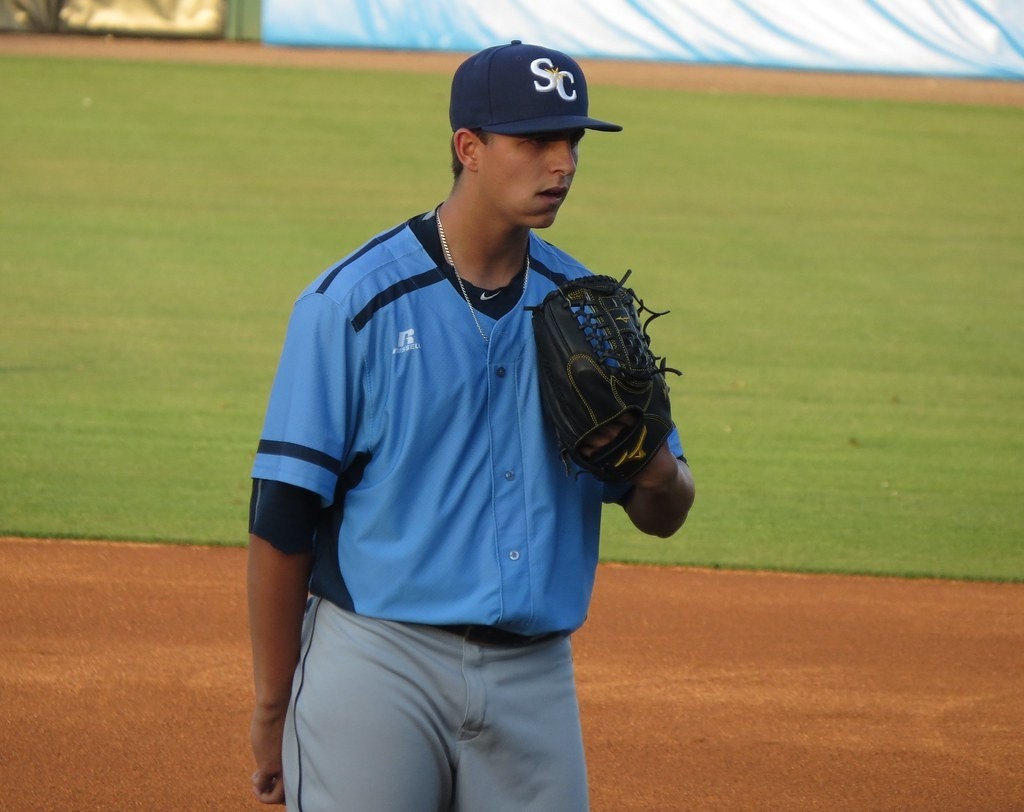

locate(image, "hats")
(449, 40), (623, 136)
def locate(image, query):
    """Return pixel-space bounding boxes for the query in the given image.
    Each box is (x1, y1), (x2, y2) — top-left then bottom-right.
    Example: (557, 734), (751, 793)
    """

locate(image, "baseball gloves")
(532, 272), (674, 481)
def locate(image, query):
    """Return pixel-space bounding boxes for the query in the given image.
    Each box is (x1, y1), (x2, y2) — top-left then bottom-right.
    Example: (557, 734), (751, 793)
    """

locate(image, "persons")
(250, 39), (696, 812)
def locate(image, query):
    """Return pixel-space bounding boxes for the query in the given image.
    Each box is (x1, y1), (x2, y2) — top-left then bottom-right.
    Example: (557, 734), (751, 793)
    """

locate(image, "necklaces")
(436, 206), (532, 342)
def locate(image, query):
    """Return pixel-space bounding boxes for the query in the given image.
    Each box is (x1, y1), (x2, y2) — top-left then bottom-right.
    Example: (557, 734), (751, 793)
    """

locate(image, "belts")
(434, 625), (569, 647)
